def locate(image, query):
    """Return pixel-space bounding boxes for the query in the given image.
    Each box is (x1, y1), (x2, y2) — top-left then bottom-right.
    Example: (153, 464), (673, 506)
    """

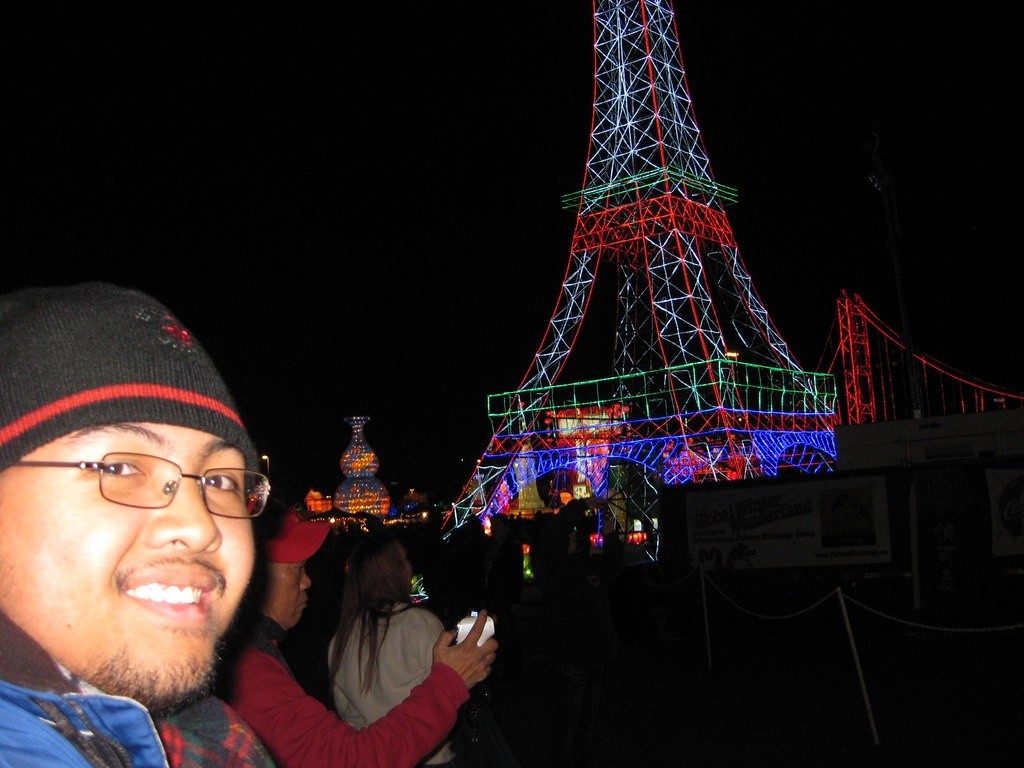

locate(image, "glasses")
(13, 451), (271, 520)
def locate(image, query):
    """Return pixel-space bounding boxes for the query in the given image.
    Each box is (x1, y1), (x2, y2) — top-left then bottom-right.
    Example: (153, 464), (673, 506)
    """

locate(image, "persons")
(0, 281), (284, 768)
(532, 523), (621, 768)
(329, 532), (463, 768)
(211, 489), (499, 768)
(486, 516), (522, 685)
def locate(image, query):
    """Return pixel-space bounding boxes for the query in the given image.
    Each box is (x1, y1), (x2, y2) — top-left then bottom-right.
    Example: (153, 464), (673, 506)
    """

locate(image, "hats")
(0, 287), (259, 494)
(246, 493), (330, 564)
